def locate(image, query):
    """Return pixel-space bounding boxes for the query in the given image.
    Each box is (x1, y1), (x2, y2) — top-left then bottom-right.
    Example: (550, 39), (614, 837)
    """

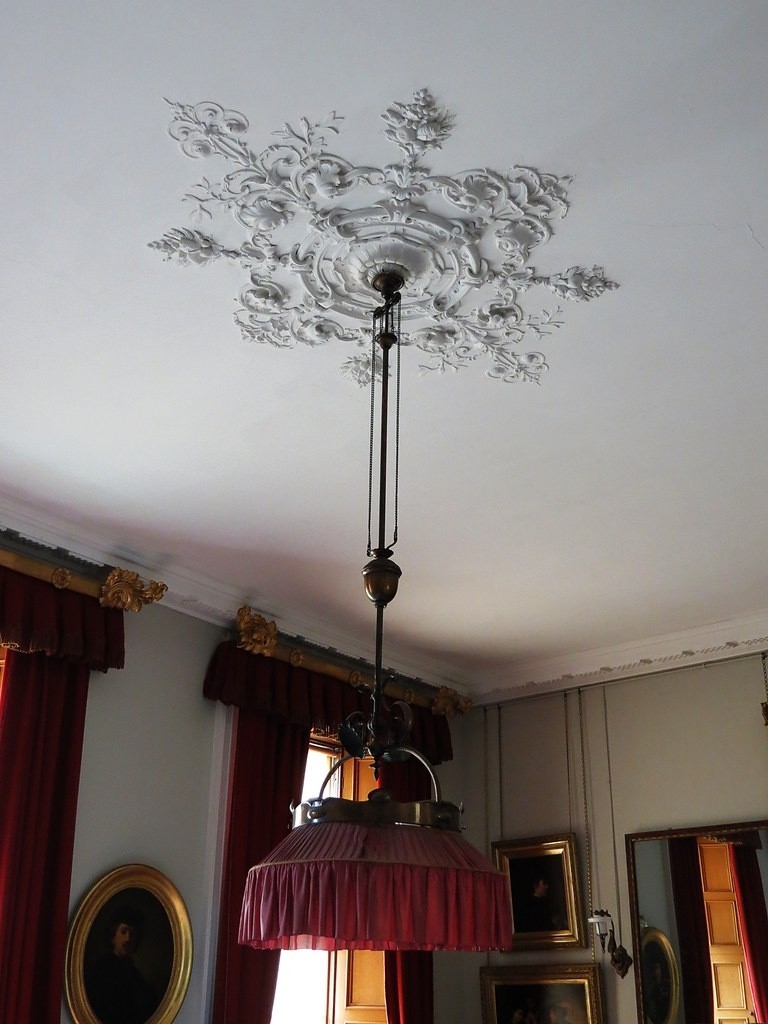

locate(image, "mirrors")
(623, 819), (768, 1024)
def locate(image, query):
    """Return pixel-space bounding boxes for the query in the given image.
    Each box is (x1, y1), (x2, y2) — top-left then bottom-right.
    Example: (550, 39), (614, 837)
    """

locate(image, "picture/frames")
(487, 835), (586, 949)
(480, 963), (605, 1024)
(640, 927), (680, 1024)
(63, 866), (191, 1024)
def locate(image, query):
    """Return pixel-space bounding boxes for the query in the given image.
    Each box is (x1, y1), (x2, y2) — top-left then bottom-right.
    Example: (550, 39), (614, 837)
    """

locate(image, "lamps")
(587, 911), (632, 978)
(236, 273), (514, 948)
